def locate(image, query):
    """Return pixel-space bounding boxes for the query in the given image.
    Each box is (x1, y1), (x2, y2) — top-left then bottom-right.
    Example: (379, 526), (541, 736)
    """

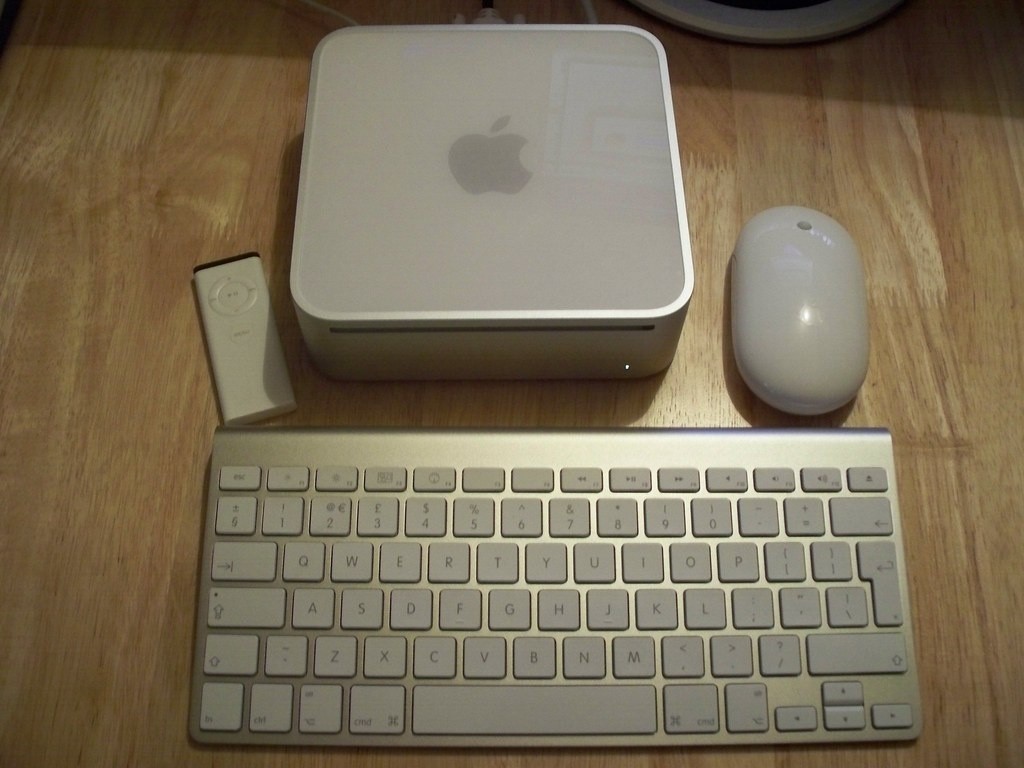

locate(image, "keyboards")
(186, 426), (923, 746)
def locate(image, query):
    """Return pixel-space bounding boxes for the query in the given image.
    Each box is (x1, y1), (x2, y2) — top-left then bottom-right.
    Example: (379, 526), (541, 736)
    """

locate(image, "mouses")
(730, 204), (868, 417)
